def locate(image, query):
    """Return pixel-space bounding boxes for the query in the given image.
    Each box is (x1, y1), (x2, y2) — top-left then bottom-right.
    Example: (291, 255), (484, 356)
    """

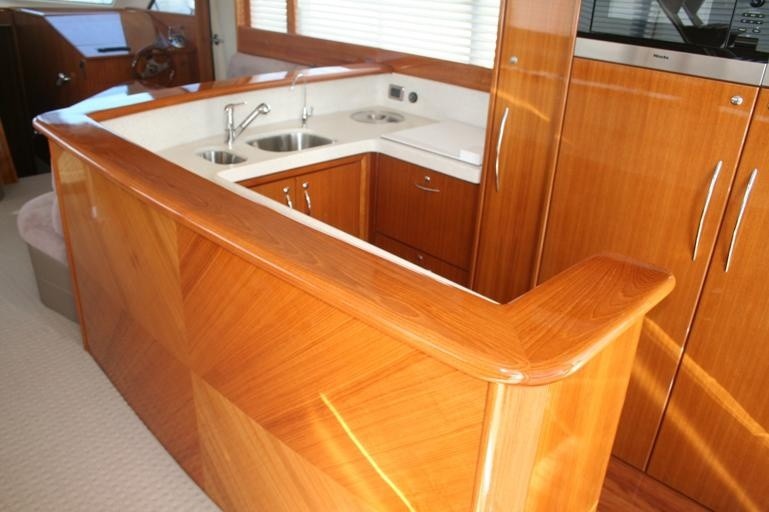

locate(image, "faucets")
(290, 73), (312, 126)
(222, 101), (272, 147)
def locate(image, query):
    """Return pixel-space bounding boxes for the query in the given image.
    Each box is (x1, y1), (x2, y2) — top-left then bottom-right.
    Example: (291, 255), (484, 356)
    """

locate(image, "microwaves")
(574, 0), (768, 91)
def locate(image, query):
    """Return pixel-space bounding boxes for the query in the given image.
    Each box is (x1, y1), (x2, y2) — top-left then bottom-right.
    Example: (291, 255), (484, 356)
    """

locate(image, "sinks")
(198, 149), (246, 166)
(245, 131), (332, 153)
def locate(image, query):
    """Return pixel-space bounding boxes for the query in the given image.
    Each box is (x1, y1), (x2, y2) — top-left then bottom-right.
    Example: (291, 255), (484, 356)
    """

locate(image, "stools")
(1, 113), (23, 202)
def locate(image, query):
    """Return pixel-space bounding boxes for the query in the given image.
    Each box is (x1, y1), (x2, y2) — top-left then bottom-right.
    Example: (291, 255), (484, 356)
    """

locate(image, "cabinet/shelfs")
(237, 154), (368, 242)
(468, 0), (581, 306)
(537, 57), (768, 511)
(371, 151), (479, 285)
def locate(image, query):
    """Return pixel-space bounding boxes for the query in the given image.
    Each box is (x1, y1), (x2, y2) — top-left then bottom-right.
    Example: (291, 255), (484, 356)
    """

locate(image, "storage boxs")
(17, 192), (79, 326)
